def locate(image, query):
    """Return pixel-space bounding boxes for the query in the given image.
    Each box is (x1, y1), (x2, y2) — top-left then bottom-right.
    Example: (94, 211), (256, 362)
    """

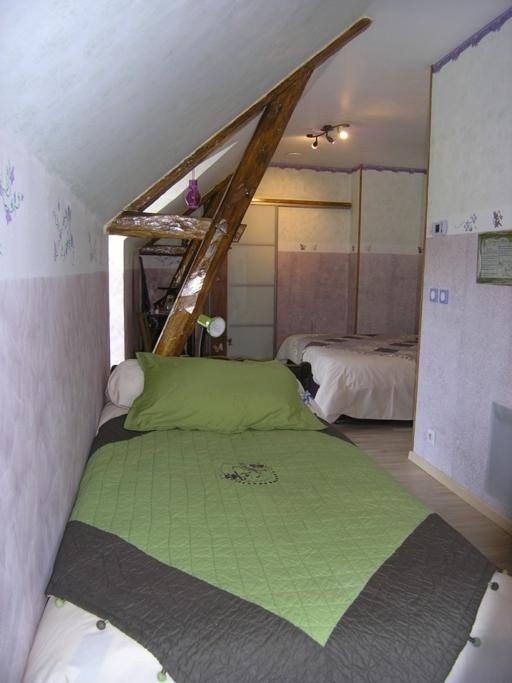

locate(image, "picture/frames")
(476, 231), (511, 286)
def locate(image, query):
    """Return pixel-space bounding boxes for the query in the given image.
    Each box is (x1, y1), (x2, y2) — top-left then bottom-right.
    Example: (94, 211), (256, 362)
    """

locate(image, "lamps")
(185, 168), (201, 210)
(195, 313), (225, 357)
(306, 123), (350, 149)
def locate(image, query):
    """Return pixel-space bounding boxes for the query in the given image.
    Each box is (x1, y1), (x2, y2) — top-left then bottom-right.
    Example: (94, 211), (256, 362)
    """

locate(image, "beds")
(275, 333), (418, 424)
(20, 352), (512, 683)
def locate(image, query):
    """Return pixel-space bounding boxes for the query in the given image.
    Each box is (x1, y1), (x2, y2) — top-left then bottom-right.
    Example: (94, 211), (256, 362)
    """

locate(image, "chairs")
(139, 313), (185, 354)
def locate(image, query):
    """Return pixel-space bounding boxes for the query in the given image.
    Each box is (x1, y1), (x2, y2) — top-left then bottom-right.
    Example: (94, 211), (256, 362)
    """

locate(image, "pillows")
(105, 352), (329, 435)
